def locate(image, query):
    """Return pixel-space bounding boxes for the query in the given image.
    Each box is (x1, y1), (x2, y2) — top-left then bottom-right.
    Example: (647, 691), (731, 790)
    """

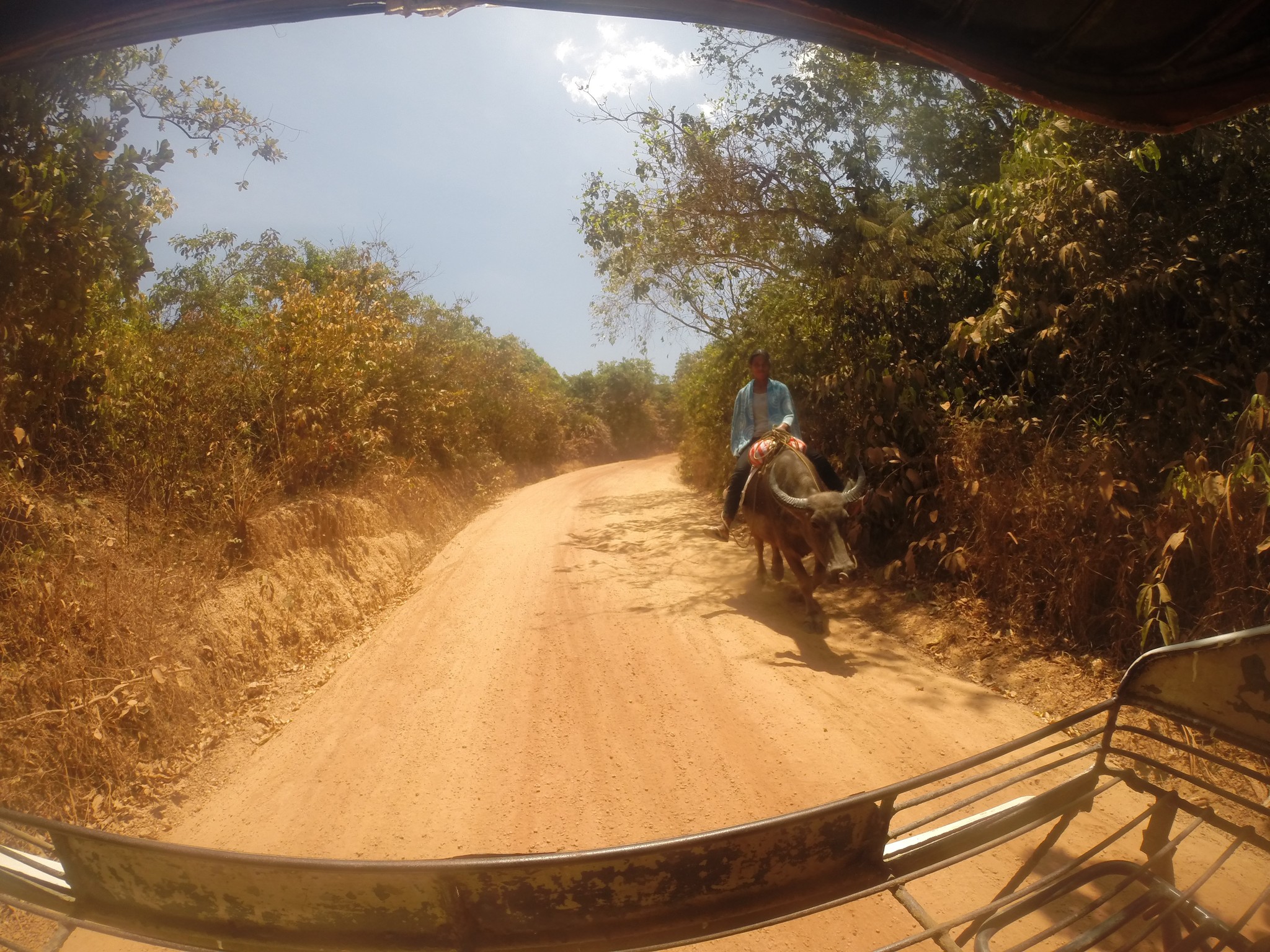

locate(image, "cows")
(739, 449), (868, 617)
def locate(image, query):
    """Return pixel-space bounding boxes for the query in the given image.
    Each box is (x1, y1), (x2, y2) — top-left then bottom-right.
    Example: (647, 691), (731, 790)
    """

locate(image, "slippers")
(702, 526), (729, 544)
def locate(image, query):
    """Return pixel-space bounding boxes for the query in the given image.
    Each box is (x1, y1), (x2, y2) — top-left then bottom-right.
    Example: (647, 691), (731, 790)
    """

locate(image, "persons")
(702, 348), (844, 542)
